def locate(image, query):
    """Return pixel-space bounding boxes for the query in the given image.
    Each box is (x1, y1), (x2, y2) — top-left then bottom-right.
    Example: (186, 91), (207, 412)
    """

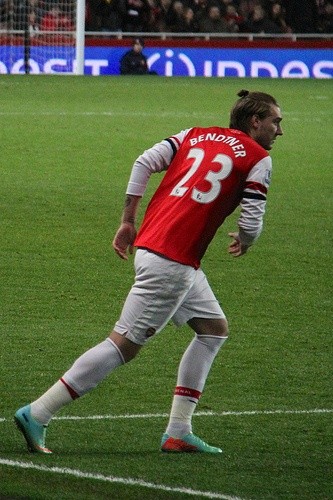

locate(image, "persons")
(15, 91), (283, 454)
(0, 0), (332, 42)
(120, 39), (157, 76)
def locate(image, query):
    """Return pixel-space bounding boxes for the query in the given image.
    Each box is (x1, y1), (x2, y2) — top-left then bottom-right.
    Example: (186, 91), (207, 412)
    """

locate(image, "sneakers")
(13, 404), (53, 455)
(160, 432), (223, 454)
